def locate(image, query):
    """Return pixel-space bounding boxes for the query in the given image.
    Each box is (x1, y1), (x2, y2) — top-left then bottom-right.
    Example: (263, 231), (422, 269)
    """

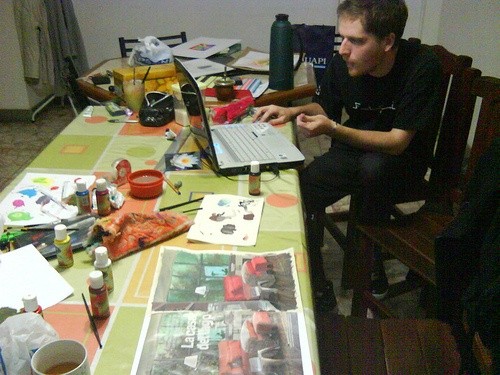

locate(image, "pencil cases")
(139, 90), (175, 127)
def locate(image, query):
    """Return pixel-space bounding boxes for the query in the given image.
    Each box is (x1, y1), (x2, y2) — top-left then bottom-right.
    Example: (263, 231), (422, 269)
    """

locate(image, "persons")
(251, 0), (439, 315)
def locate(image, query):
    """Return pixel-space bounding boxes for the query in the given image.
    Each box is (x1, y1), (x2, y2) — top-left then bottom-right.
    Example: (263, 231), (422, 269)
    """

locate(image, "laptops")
(174, 58), (306, 176)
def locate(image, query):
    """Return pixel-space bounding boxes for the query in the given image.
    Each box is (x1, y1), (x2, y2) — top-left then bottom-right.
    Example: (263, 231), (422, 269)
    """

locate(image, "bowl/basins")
(127, 170), (164, 199)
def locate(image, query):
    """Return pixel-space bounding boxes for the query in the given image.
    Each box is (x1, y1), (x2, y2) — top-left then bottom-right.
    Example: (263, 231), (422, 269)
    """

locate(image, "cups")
(30, 339), (90, 375)
(214, 80), (235, 104)
(123, 80), (145, 113)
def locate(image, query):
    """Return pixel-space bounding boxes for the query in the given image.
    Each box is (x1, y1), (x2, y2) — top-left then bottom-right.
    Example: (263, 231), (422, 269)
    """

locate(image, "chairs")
(317, 39), (473, 290)
(351, 68), (500, 320)
(118, 31), (187, 58)
(315, 312), (500, 375)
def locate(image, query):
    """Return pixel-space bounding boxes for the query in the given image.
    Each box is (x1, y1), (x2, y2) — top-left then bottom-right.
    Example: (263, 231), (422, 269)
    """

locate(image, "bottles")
(75, 179), (92, 216)
(96, 178), (110, 217)
(268, 14), (294, 91)
(22, 293), (44, 322)
(93, 246), (114, 294)
(53, 224), (74, 268)
(88, 271), (110, 319)
(248, 162), (262, 195)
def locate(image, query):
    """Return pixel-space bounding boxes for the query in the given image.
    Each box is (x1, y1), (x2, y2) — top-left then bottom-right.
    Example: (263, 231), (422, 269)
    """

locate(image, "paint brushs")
(159, 192), (215, 211)
(141, 66), (152, 83)
(162, 176), (180, 195)
(81, 293), (102, 348)
(20, 227), (77, 233)
(133, 68), (135, 84)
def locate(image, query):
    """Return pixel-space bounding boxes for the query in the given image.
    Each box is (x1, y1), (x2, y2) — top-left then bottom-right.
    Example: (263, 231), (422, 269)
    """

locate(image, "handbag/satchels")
(128, 36), (172, 67)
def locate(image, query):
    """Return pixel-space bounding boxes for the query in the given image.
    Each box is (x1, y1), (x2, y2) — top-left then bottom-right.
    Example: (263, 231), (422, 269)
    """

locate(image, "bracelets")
(330, 121), (340, 137)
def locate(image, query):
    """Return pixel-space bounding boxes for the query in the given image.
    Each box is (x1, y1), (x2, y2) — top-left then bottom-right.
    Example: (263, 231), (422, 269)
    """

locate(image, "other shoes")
(368, 258), (389, 301)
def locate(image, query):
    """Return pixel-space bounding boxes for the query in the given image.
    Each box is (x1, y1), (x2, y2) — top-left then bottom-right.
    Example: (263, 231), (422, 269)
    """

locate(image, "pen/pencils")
(0, 214), (100, 259)
(241, 78), (261, 91)
(108, 119), (140, 123)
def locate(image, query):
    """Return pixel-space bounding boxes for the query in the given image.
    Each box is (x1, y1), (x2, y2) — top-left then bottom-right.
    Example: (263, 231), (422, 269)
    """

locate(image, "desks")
(0, 48), (320, 375)
